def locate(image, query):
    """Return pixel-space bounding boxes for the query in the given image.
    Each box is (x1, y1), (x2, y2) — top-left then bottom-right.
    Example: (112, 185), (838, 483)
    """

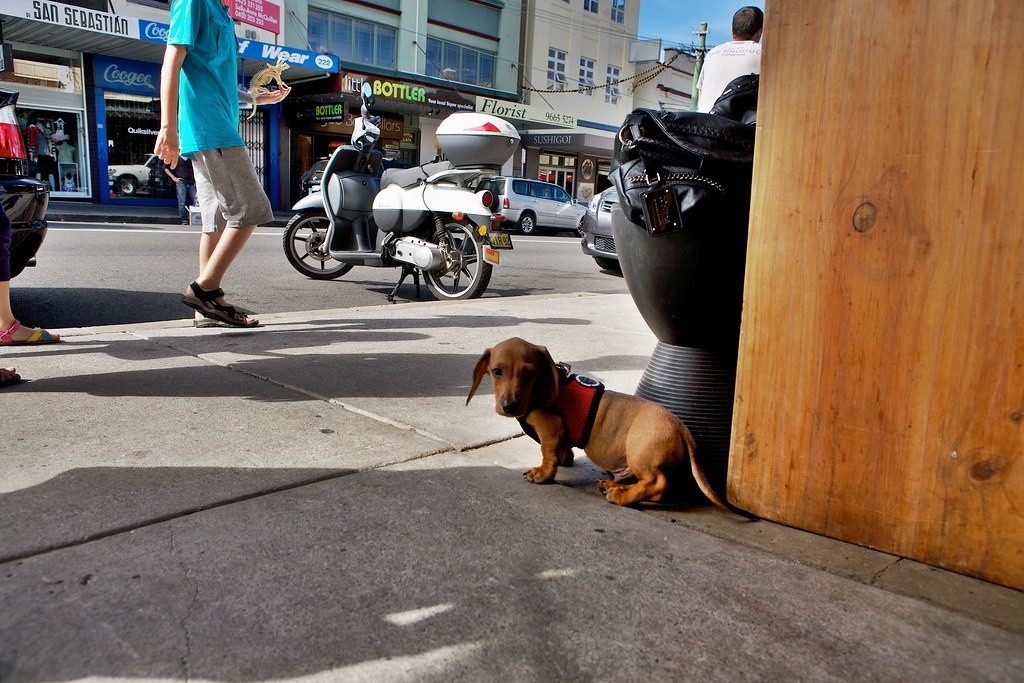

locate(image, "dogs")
(464, 337), (730, 514)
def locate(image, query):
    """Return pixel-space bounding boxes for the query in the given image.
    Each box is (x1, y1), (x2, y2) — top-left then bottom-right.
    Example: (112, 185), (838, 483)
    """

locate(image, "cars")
(0, 84), (50, 284)
(298, 160), (330, 228)
(581, 184), (621, 273)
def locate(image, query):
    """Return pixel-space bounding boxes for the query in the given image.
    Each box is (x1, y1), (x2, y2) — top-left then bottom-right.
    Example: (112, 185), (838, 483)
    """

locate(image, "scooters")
(281, 82), (521, 303)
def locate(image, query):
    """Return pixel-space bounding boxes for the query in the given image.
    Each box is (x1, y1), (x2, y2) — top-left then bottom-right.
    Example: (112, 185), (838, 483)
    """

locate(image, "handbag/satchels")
(610, 74), (761, 235)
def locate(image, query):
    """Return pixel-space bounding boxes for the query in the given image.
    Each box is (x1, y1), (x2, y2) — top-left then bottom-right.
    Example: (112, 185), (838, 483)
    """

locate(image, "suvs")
(483, 175), (589, 239)
(106, 153), (175, 197)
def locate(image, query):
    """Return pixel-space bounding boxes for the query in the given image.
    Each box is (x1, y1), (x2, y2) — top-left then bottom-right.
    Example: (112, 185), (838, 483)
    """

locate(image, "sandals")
(181, 280), (259, 328)
(0, 367), (21, 388)
(0, 320), (58, 346)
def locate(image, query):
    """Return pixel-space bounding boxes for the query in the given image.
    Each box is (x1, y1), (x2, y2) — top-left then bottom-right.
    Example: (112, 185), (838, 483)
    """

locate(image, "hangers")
(26, 124), (40, 130)
(54, 117), (67, 125)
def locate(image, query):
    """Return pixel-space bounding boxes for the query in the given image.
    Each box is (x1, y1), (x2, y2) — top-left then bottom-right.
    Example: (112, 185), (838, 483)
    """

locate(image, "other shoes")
(182, 220), (190, 227)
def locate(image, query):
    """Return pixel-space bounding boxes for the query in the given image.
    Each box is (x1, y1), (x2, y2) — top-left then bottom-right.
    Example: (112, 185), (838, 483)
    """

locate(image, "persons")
(153, 0), (291, 328)
(0, 199), (61, 387)
(695, 6), (764, 125)
(23, 124), (75, 191)
(162, 148), (198, 225)
